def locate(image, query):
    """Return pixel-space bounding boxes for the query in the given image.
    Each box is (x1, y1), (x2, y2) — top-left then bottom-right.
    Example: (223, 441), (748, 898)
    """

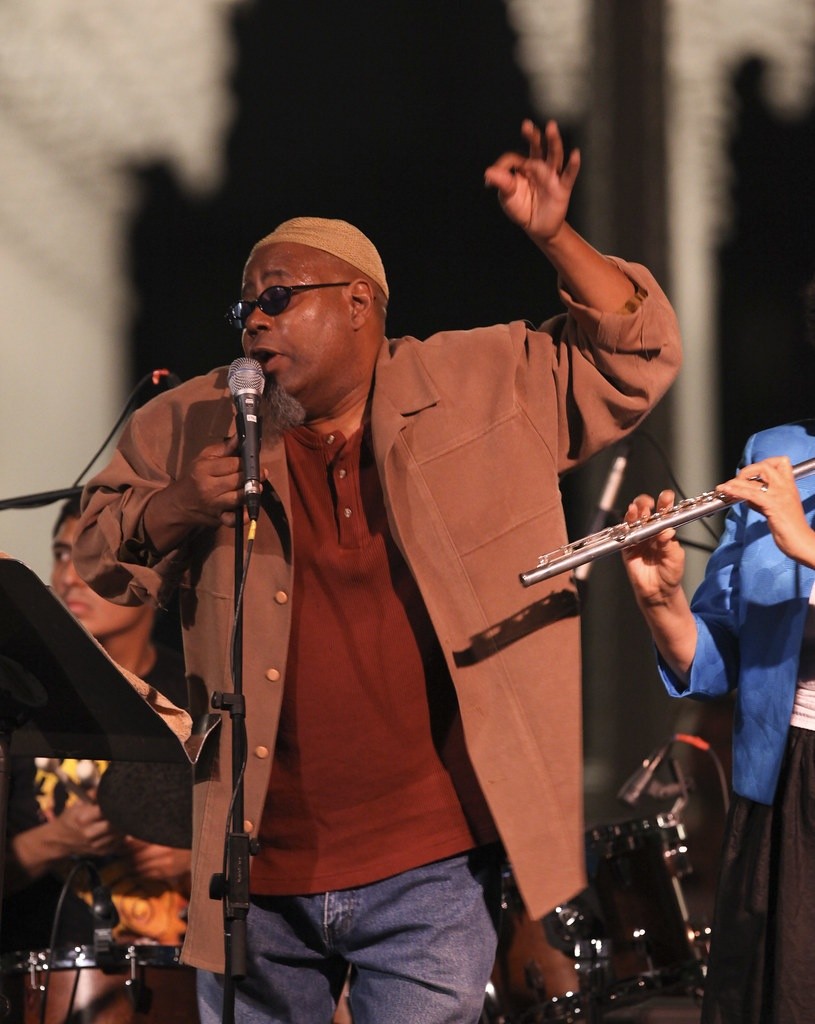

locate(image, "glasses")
(224, 282), (352, 330)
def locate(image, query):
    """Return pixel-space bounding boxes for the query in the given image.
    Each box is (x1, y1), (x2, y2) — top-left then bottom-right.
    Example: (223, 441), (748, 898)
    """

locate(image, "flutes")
(518, 453), (815, 585)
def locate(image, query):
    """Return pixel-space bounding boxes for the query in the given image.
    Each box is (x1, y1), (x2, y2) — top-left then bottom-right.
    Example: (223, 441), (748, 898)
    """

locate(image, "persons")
(7, 497), (189, 947)
(75, 117), (680, 1024)
(623, 276), (815, 1024)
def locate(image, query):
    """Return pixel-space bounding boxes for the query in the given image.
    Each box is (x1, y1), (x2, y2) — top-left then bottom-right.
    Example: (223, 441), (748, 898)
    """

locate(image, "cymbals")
(95, 732), (203, 851)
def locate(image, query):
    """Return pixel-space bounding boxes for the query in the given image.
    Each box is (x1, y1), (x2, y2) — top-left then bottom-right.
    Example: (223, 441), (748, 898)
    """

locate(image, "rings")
(761, 484), (767, 492)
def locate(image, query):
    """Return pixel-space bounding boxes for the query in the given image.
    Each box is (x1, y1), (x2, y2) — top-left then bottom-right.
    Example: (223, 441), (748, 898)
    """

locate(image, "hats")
(252, 217), (393, 301)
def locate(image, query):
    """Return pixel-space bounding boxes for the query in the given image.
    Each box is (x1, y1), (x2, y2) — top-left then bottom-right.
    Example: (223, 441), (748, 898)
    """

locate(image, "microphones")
(574, 436), (634, 581)
(616, 737), (675, 807)
(227, 357), (266, 517)
(91, 874), (119, 930)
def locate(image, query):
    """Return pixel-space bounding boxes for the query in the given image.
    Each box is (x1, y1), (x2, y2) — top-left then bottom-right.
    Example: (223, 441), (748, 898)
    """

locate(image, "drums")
(464, 813), (711, 1023)
(0, 938), (208, 1024)
